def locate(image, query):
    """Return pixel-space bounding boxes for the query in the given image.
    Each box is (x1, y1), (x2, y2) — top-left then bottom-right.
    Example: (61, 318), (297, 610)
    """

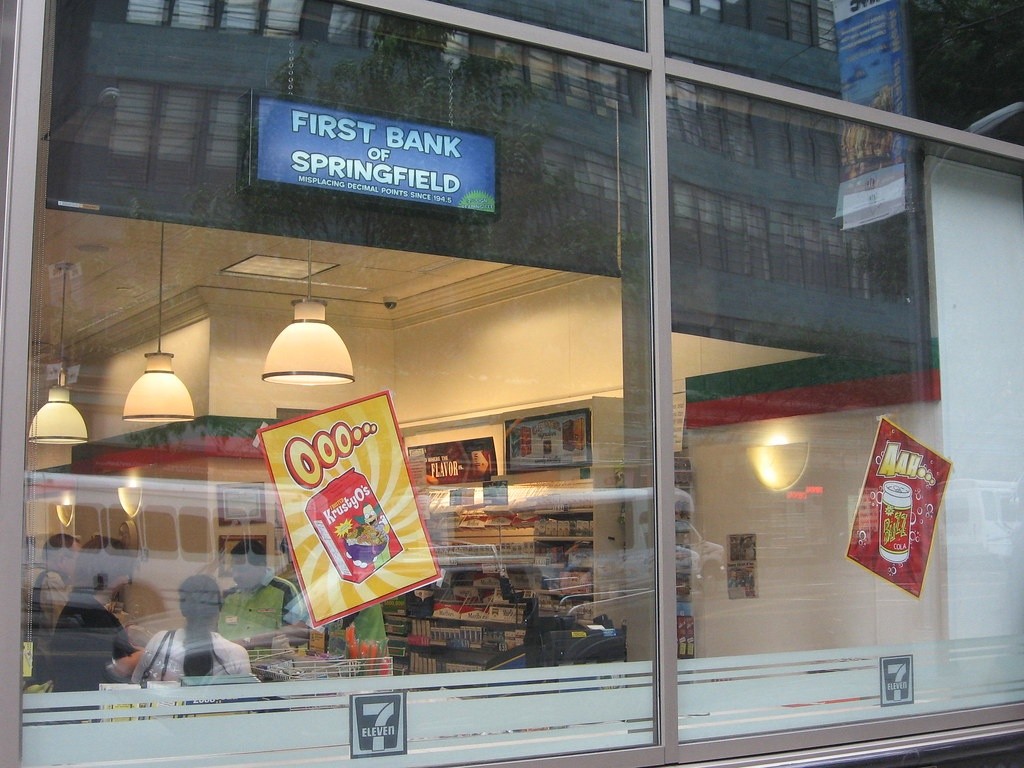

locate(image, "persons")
(32, 533), (77, 682)
(132, 574), (251, 682)
(306, 602), (388, 655)
(50, 537), (143, 691)
(219, 537), (297, 659)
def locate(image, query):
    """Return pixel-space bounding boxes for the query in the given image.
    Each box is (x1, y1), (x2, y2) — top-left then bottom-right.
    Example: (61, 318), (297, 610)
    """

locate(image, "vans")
(942, 479), (1019, 558)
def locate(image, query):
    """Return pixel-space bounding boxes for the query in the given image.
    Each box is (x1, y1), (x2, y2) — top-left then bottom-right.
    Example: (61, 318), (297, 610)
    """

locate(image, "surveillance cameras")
(382, 297), (397, 309)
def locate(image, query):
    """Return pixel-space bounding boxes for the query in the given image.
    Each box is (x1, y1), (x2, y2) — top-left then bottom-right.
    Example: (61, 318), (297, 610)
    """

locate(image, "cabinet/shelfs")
(614, 452), (690, 657)
(373, 396), (624, 673)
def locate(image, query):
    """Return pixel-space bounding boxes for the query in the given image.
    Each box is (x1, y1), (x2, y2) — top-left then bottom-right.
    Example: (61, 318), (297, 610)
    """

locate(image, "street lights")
(918, 102), (1024, 189)
(62, 86), (121, 183)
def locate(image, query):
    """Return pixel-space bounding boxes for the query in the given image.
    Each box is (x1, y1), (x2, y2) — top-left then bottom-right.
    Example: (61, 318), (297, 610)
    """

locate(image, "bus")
(22, 469), (731, 633)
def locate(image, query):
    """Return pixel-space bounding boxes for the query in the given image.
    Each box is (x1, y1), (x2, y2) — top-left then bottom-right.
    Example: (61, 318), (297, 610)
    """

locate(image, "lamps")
(123, 223), (196, 423)
(746, 440), (809, 492)
(56, 505), (73, 526)
(117, 486), (142, 518)
(261, 240), (355, 385)
(29, 263), (88, 444)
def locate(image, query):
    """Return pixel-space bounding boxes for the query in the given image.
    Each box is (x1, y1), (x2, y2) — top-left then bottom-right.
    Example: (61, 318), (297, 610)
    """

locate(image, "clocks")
(119, 521), (138, 551)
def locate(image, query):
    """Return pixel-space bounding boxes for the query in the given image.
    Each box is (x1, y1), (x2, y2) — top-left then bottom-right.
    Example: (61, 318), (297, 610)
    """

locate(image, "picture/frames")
(218, 534), (267, 577)
(216, 482), (266, 526)
